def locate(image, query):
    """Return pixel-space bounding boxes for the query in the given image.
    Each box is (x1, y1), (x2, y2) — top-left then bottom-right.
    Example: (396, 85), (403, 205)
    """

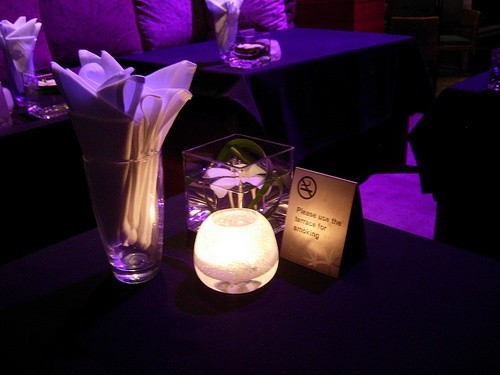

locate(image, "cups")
(84, 148), (165, 286)
(24, 71), (69, 119)
(229, 33), (271, 67)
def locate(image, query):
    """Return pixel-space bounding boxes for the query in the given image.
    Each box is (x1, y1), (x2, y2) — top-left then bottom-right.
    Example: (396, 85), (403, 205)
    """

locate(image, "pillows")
(0, 0), (296, 78)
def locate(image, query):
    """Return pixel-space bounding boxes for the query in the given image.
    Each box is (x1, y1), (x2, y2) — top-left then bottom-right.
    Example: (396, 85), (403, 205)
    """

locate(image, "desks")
(406, 67), (500, 264)
(119, 27), (412, 181)
(0, 67), (81, 137)
(0, 191), (500, 375)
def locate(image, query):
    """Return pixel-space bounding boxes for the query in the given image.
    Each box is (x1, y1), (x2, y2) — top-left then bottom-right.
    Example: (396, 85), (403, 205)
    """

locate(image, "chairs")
(386, 0), (481, 57)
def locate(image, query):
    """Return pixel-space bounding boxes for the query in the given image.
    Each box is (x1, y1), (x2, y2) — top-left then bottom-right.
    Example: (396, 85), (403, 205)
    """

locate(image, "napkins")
(0, 16), (43, 78)
(51, 48), (197, 251)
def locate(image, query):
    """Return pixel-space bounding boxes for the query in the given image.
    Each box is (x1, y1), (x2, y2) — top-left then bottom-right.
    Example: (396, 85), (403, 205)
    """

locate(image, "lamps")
(193, 208), (280, 294)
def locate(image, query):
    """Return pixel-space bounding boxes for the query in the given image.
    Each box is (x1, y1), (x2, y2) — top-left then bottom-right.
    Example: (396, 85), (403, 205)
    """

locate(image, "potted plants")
(182, 134), (294, 235)
